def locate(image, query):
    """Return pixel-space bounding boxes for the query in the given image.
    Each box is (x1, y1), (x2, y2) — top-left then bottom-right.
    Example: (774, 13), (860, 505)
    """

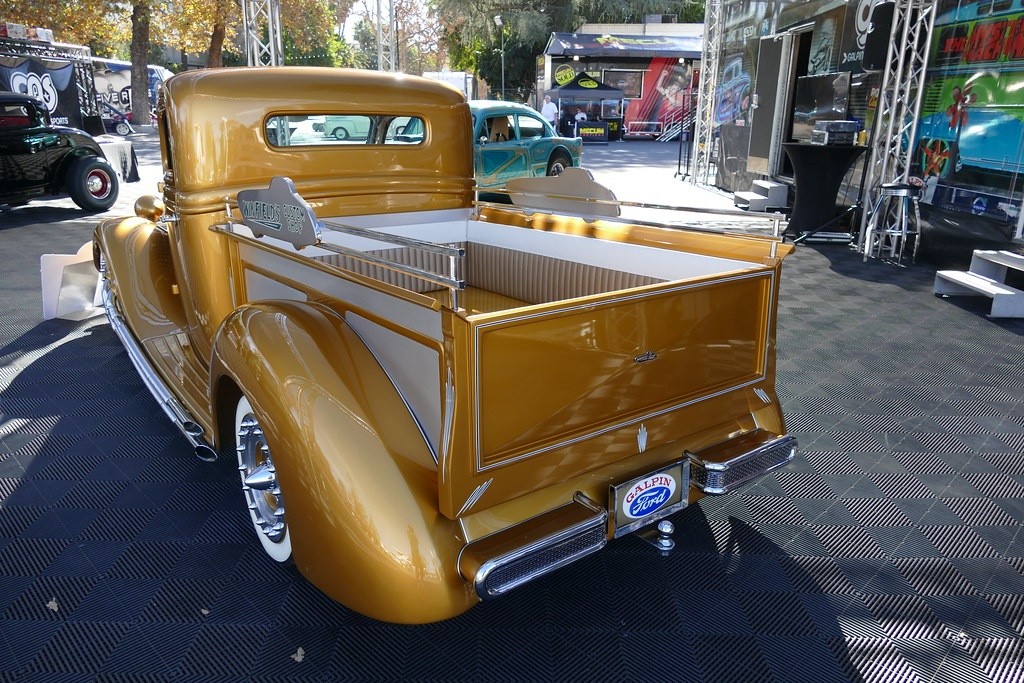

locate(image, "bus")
(899, 1), (1024, 183)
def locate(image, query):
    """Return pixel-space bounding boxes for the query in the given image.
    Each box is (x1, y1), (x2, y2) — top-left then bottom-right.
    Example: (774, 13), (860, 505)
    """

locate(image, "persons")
(574, 106), (588, 122)
(542, 94), (559, 127)
(618, 101), (626, 139)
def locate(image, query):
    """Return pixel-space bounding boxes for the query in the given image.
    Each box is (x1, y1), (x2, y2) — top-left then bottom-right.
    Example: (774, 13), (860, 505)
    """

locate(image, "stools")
(861, 182), (921, 263)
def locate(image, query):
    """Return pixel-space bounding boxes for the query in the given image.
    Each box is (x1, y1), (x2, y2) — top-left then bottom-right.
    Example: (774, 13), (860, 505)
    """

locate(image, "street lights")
(494, 15), (506, 100)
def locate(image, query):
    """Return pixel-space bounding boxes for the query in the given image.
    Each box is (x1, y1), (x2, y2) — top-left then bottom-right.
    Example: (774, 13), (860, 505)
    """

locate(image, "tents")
(544, 71), (624, 141)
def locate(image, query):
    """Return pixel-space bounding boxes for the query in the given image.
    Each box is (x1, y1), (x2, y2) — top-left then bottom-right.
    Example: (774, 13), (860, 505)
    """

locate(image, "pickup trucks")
(90, 64), (804, 628)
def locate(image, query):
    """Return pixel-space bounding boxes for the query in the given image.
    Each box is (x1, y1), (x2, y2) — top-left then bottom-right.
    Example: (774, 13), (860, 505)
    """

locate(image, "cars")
(466, 97), (583, 193)
(324, 112), (420, 140)
(0, 92), (119, 211)
(98, 102), (134, 135)
